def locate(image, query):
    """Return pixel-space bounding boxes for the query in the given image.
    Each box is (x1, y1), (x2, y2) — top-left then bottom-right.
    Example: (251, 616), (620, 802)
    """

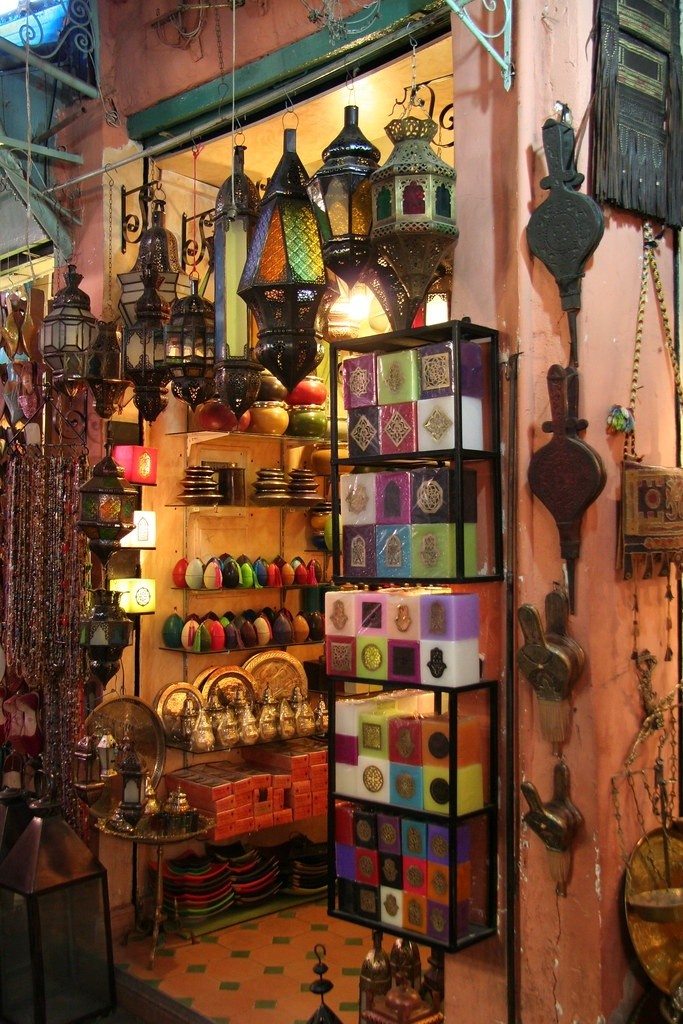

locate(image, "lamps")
(77, 429), (136, 567)
(78, 582), (134, 691)
(114, 445), (158, 487)
(41, 105), (459, 407)
(120, 509), (157, 550)
(109, 577), (156, 615)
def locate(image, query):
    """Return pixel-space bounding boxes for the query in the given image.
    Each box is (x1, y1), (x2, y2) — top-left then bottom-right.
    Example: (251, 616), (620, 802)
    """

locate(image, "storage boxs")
(339, 468), (477, 578)
(325, 588), (487, 933)
(343, 344), (484, 458)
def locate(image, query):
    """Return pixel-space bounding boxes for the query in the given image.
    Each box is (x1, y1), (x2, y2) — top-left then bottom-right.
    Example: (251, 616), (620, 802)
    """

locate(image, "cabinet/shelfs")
(163, 431), (331, 849)
(327, 321), (505, 954)
